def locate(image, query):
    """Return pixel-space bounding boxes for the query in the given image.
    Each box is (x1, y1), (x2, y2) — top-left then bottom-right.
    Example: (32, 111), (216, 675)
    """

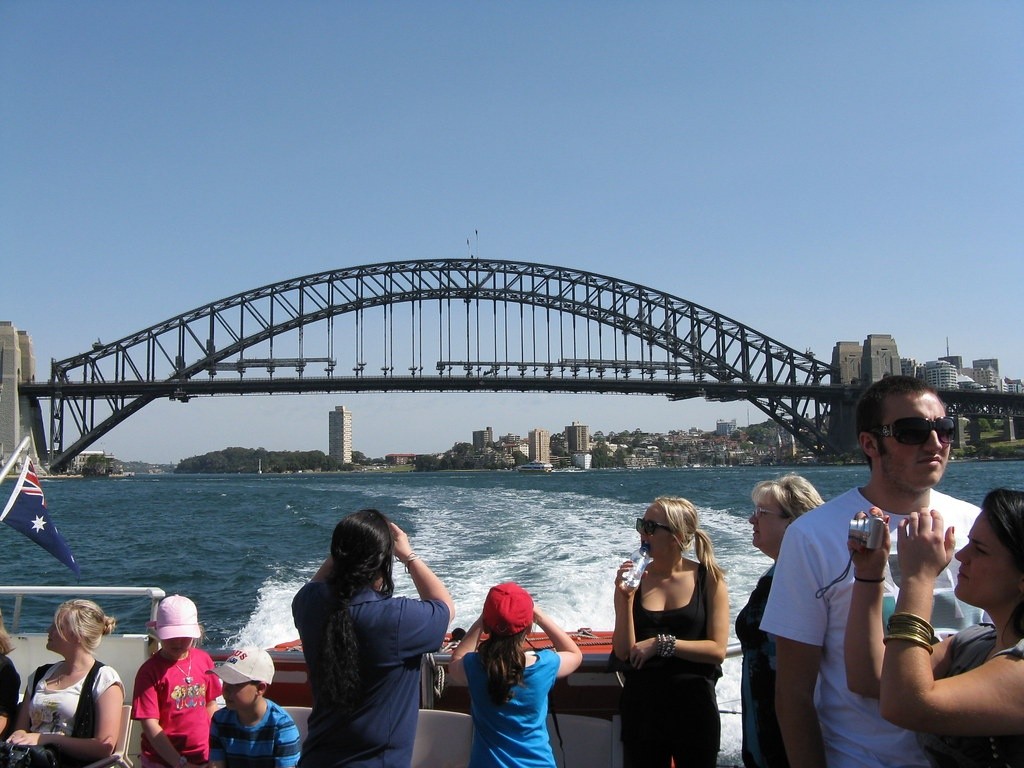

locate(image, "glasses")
(752, 506), (790, 518)
(636, 517), (671, 536)
(868, 416), (955, 446)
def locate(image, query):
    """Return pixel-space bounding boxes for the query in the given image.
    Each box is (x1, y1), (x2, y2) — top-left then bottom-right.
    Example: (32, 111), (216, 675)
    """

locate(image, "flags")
(0, 454), (82, 585)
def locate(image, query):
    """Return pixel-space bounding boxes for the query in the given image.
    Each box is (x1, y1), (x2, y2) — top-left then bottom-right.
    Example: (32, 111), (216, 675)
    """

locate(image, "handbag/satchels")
(0, 741), (60, 768)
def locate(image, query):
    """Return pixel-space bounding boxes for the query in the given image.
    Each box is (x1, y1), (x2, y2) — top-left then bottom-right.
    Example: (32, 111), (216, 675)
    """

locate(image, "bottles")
(621, 542), (651, 588)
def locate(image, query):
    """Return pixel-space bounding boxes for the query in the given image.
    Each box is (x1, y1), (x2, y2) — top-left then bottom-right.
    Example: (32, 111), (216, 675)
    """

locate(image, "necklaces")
(161, 645), (192, 684)
(55, 659), (95, 686)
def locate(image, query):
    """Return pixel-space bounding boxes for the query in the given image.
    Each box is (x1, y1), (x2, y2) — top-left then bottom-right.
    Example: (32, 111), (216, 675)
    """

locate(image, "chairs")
(81, 705), (135, 768)
(409, 709), (473, 768)
(546, 713), (624, 768)
(281, 707), (311, 741)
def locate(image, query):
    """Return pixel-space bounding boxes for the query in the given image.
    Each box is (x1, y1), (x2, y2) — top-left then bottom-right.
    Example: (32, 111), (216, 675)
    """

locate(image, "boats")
(518, 459), (553, 473)
(265, 625), (624, 722)
(551, 466), (588, 474)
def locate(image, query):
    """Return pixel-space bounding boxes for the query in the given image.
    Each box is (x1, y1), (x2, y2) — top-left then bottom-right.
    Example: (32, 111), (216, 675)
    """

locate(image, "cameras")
(848, 515), (884, 550)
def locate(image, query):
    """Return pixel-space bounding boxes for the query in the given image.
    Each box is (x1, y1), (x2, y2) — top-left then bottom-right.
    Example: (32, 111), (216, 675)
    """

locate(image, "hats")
(482, 582), (535, 636)
(145, 594), (201, 640)
(205, 646), (275, 686)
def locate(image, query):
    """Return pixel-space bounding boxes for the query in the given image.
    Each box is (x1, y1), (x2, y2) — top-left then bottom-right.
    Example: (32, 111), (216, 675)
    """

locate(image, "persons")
(131, 594), (224, 768)
(734, 474), (826, 768)
(447, 581), (583, 768)
(759, 375), (995, 768)
(0, 605), (21, 768)
(208, 646), (301, 768)
(844, 486), (1024, 768)
(292, 508), (455, 768)
(612, 496), (728, 768)
(5, 598), (126, 768)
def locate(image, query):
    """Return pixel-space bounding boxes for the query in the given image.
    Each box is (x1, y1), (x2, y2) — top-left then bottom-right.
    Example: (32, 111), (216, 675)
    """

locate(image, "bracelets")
(404, 552), (421, 574)
(654, 634), (676, 658)
(853, 574), (886, 586)
(883, 612), (935, 656)
